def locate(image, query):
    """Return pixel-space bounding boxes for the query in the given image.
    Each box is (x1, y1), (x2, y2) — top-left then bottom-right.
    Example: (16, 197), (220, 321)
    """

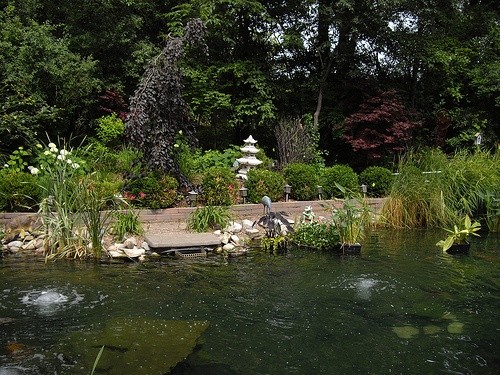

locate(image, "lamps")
(361, 184), (369, 198)
(239, 186), (249, 204)
(284, 184), (291, 202)
(316, 185), (322, 200)
(189, 190), (197, 207)
